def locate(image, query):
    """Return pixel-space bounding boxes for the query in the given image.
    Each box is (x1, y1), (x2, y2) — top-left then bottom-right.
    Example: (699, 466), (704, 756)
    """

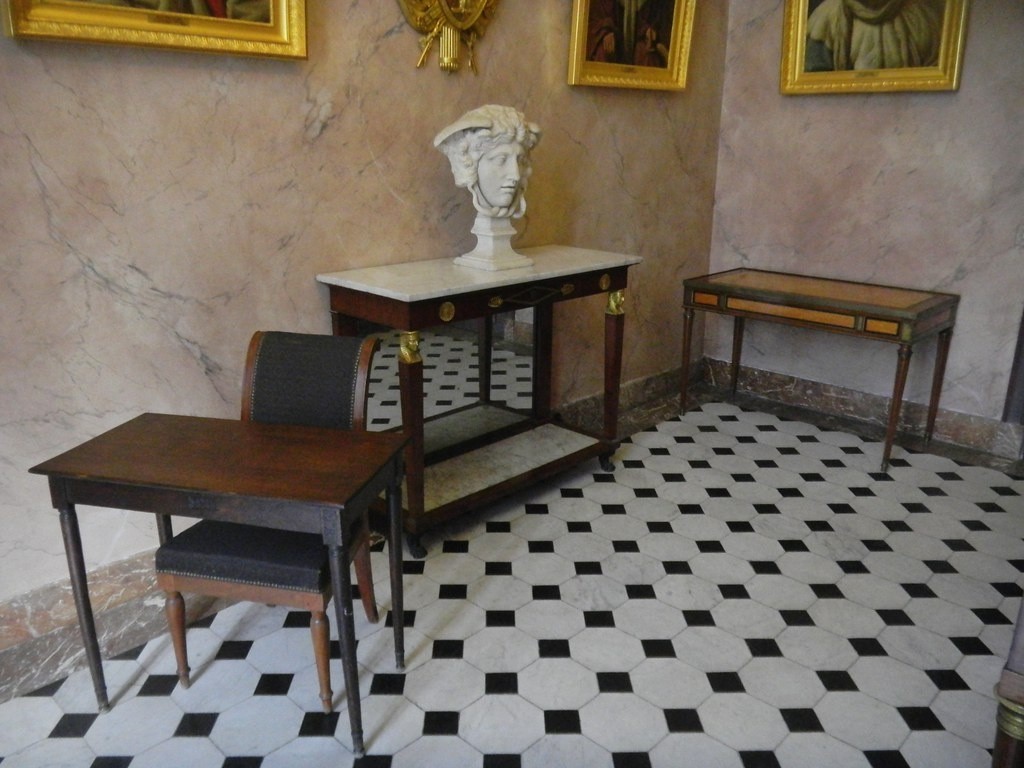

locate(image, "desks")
(27, 413), (415, 761)
(318, 244), (643, 550)
(678, 267), (962, 474)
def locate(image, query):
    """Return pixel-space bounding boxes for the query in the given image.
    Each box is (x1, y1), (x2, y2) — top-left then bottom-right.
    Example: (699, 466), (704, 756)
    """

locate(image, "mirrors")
(367, 303), (553, 473)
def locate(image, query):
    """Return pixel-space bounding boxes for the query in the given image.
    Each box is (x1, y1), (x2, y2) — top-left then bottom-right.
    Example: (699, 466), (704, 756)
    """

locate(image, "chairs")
(155, 331), (380, 713)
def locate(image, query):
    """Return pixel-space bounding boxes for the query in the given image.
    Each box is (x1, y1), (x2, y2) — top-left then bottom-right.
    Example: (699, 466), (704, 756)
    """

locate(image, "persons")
(587, 0), (668, 67)
(434, 103), (543, 218)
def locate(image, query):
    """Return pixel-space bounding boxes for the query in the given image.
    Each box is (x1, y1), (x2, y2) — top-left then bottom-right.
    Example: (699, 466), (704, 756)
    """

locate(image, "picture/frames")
(779, 0), (971, 97)
(568, 0), (696, 92)
(2, 0), (309, 61)
